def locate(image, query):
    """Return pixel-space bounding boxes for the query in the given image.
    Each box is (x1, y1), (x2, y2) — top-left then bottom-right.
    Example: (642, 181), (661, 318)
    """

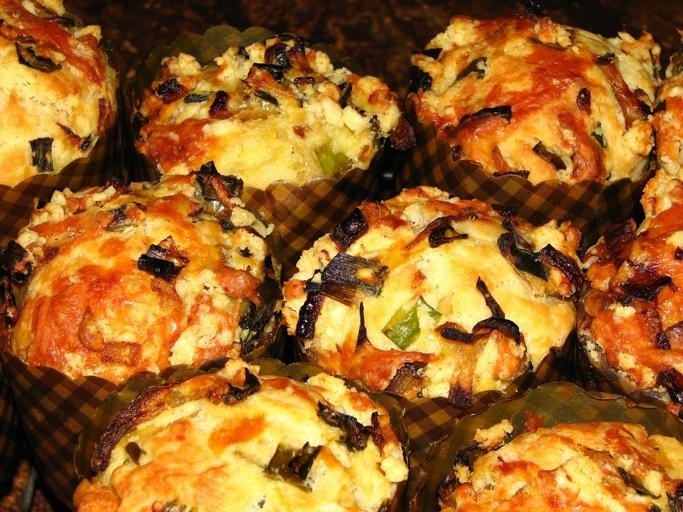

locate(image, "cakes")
(582, 172), (683, 397)
(0, 0), (117, 189)
(281, 184), (582, 402)
(132, 32), (400, 193)
(441, 419), (683, 512)
(409, 9), (656, 182)
(7, 164), (267, 382)
(656, 34), (683, 172)
(74, 360), (408, 512)
